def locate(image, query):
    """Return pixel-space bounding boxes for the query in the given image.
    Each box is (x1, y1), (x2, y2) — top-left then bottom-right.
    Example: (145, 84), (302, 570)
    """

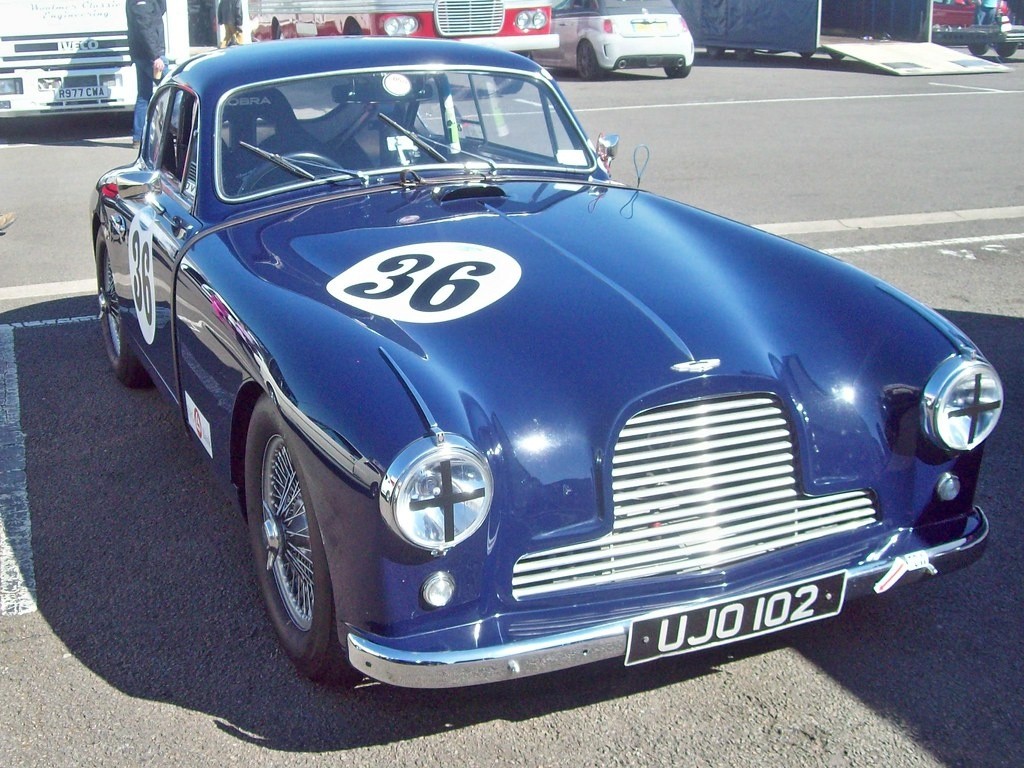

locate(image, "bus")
(250, 1), (562, 94)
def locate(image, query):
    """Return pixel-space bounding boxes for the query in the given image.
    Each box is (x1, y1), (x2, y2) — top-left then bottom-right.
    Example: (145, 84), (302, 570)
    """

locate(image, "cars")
(529, 0), (696, 81)
(86, 32), (1006, 690)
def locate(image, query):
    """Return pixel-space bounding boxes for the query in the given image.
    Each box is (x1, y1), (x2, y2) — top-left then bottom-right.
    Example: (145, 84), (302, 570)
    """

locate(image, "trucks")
(672, 0), (1018, 77)
(0, 0), (252, 129)
(931, 0), (1024, 58)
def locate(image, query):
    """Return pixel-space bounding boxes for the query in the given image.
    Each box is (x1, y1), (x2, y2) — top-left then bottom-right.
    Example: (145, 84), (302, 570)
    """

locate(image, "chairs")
(343, 129), (401, 170)
